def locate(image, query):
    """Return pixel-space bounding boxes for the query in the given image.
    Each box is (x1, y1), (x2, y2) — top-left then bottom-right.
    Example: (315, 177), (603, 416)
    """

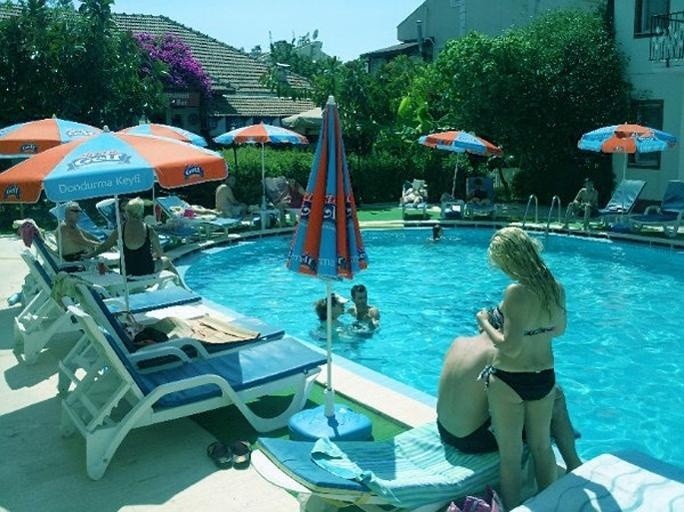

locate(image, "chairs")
(95, 191), (297, 249)
(628, 179), (684, 238)
(591, 179), (648, 230)
(4, 202), (203, 367)
(509, 447), (684, 512)
(248, 406), (549, 512)
(465, 176), (497, 221)
(58, 278), (332, 477)
(400, 178), (429, 218)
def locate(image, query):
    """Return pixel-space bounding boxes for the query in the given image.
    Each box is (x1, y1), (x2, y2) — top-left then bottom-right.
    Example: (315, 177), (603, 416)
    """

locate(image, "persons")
(436, 326), (580, 454)
(474, 227), (567, 511)
(215, 174), (248, 230)
(79, 195), (186, 289)
(345, 284), (380, 351)
(307, 293), (350, 348)
(560, 177), (599, 231)
(465, 178), (491, 206)
(111, 195), (186, 232)
(399, 181), (428, 208)
(271, 175), (306, 228)
(54, 203), (118, 262)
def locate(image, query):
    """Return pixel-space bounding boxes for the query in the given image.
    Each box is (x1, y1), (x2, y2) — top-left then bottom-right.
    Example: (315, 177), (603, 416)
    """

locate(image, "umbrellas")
(577, 122), (677, 180)
(284, 96), (369, 419)
(0, 126), (230, 313)
(213, 121), (311, 209)
(115, 124), (209, 224)
(418, 129), (503, 196)
(0, 114), (105, 266)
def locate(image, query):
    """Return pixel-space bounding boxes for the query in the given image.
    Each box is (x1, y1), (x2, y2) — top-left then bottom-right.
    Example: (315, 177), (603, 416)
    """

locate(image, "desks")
(441, 199), (466, 217)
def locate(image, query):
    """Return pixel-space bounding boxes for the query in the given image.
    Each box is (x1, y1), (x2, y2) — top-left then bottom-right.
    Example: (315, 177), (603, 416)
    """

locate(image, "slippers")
(208, 441), (232, 468)
(230, 441), (252, 469)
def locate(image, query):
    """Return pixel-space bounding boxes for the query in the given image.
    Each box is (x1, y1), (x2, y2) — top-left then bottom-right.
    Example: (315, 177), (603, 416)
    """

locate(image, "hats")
(334, 294), (348, 305)
